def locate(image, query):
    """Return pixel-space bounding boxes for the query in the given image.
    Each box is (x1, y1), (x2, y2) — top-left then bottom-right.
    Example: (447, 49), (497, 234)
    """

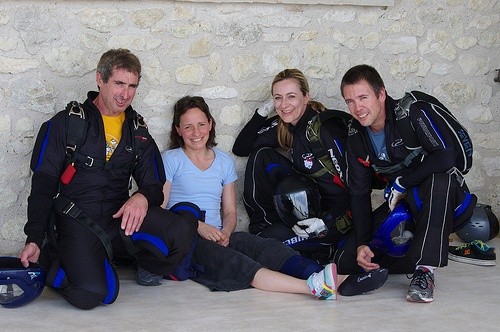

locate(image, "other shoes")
(136, 264), (163, 285)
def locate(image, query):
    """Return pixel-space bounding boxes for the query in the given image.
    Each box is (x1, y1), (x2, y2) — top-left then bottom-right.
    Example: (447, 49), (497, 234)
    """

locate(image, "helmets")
(0, 255), (46, 308)
(455, 204), (500, 243)
(369, 205), (414, 258)
(271, 173), (320, 228)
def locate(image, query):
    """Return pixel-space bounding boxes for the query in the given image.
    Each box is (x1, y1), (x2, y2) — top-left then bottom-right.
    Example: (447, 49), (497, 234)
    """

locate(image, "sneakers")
(406, 267), (435, 302)
(448, 239), (497, 265)
(307, 263), (338, 300)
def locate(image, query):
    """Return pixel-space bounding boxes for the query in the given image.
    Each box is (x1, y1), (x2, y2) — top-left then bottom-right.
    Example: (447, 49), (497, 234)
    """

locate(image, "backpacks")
(345, 90), (472, 182)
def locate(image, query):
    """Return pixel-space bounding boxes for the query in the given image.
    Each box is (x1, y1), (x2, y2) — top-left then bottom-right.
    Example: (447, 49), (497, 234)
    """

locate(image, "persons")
(231, 69), (378, 266)
(328, 64), (479, 304)
(18, 48), (197, 311)
(159, 95), (390, 301)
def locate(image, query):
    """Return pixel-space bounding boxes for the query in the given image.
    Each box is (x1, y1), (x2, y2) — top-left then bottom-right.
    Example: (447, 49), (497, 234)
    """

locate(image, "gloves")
(387, 176), (406, 211)
(292, 218), (327, 238)
(258, 98), (275, 118)
